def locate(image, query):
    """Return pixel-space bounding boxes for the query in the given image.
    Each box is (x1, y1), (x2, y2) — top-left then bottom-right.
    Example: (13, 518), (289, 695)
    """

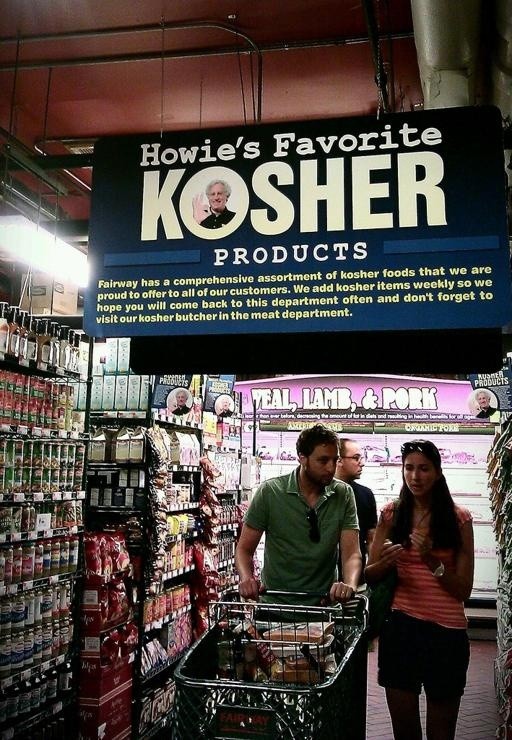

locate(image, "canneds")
(0, 437), (85, 492)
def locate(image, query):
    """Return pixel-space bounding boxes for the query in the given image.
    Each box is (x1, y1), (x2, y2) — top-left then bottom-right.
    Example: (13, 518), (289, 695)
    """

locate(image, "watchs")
(432, 562), (444, 578)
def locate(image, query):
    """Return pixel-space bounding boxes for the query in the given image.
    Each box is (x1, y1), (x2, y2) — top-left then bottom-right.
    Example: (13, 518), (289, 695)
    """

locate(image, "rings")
(342, 591), (346, 594)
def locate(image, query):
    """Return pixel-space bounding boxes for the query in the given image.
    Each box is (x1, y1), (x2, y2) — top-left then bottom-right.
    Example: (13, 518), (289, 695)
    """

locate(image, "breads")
(245, 644), (256, 662)
(285, 656), (325, 670)
(272, 662), (315, 683)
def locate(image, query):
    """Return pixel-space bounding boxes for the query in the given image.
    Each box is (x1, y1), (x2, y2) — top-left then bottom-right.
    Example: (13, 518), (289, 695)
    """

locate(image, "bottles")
(0, 369), (74, 434)
(0, 302), (82, 373)
(0, 536), (79, 584)
(0, 500), (83, 533)
(0, 581), (74, 730)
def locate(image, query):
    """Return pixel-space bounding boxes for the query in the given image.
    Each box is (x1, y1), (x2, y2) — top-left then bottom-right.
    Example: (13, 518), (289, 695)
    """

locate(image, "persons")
(191, 180), (236, 230)
(336, 438), (378, 592)
(363, 441), (474, 740)
(475, 390), (496, 419)
(235, 426), (362, 623)
(218, 398), (233, 417)
(172, 390), (191, 416)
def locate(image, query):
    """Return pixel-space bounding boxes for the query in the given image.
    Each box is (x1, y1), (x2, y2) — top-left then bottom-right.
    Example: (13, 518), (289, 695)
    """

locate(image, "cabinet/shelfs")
(0, 312), (95, 740)
(76, 373), (263, 740)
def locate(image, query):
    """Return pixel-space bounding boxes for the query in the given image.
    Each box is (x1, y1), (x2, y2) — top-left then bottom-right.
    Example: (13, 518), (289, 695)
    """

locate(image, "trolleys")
(173, 589), (369, 739)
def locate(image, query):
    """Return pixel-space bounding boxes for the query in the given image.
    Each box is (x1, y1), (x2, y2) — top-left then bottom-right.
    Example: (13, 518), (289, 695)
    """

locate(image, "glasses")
(341, 454), (364, 462)
(404, 440), (425, 452)
(306, 507), (319, 542)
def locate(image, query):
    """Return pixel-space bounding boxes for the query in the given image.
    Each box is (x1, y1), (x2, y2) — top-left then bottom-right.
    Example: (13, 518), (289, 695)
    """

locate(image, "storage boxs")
(18, 269), (79, 316)
